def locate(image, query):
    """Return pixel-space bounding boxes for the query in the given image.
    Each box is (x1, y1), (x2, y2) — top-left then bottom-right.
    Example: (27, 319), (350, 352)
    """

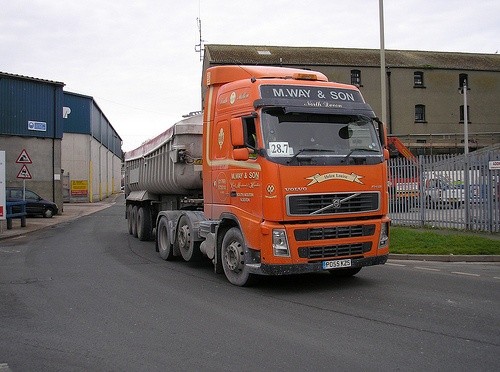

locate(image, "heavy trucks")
(124, 65), (393, 289)
(380, 136), (426, 212)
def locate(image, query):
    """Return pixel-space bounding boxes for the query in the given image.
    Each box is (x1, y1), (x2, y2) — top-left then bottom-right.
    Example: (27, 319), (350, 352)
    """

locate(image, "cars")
(5, 186), (58, 219)
